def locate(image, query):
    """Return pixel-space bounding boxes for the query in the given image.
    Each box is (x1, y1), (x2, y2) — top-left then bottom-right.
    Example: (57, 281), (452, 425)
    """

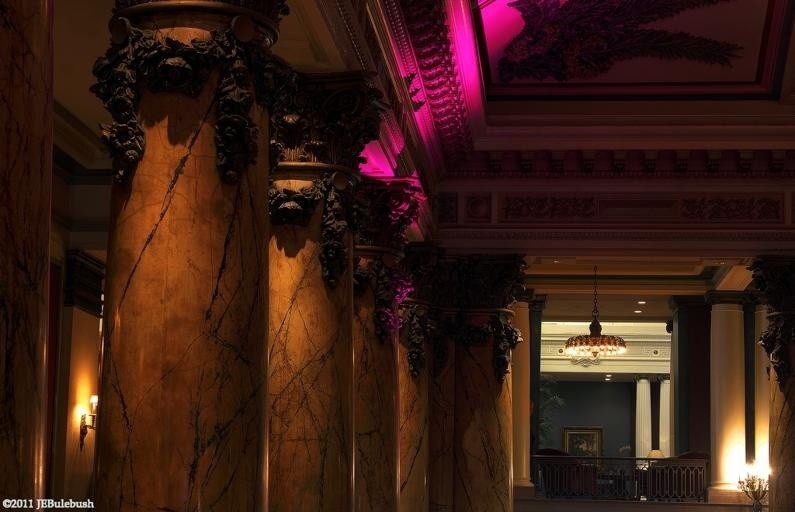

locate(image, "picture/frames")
(560, 423), (604, 469)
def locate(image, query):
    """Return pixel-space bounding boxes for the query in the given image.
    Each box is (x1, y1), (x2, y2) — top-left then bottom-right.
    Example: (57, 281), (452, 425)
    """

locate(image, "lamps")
(645, 450), (664, 460)
(562, 263), (626, 360)
(73, 391), (99, 454)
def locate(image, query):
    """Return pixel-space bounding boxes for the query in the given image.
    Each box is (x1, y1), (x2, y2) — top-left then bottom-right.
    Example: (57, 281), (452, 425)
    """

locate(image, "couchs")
(537, 447), (599, 497)
(638, 451), (710, 498)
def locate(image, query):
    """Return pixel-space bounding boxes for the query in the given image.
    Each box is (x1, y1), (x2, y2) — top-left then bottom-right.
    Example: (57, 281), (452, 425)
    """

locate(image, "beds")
(590, 469), (640, 500)
